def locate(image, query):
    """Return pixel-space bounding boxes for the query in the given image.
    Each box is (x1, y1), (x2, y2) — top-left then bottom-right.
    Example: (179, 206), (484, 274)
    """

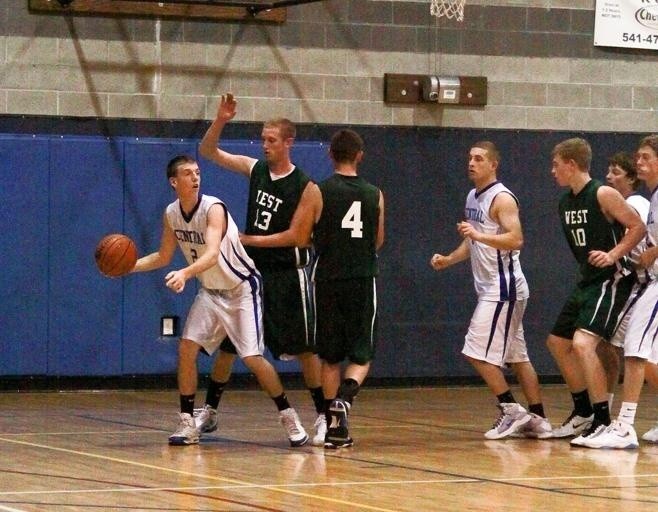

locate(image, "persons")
(596, 148), (650, 413)
(585, 131), (658, 448)
(195, 91), (329, 446)
(549, 136), (647, 448)
(104, 155), (309, 446)
(292, 128), (387, 448)
(428, 139), (553, 439)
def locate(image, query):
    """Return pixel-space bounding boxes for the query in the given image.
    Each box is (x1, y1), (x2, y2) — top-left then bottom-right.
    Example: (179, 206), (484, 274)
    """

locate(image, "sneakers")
(582, 420), (639, 451)
(311, 412), (326, 447)
(327, 395), (350, 446)
(323, 432), (353, 450)
(167, 411), (199, 445)
(483, 402), (531, 440)
(639, 422), (658, 444)
(276, 404), (309, 447)
(569, 420), (614, 448)
(551, 413), (594, 438)
(194, 403), (218, 439)
(506, 412), (552, 440)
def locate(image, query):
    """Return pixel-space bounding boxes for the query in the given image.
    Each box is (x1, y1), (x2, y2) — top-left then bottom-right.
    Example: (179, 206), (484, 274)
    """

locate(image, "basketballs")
(95, 234), (138, 277)
